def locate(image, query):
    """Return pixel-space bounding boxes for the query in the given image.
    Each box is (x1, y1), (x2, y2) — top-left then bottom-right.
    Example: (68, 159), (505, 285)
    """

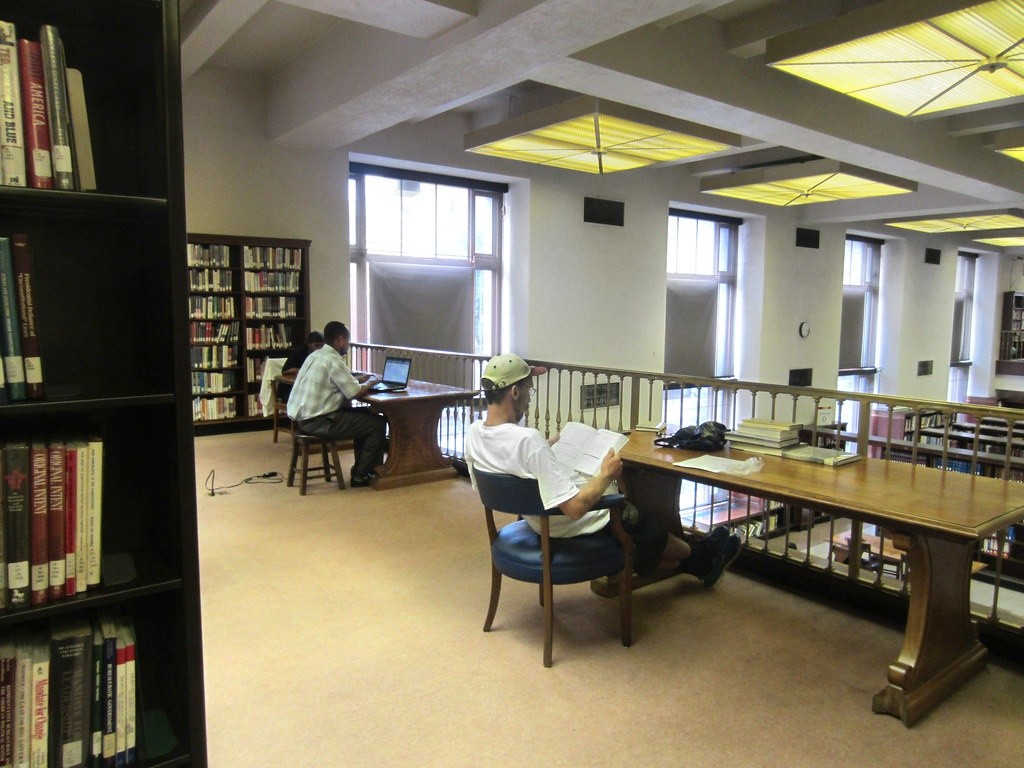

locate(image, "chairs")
(270, 382), (295, 443)
(899, 552), (911, 582)
(471, 461), (634, 668)
(848, 538), (879, 572)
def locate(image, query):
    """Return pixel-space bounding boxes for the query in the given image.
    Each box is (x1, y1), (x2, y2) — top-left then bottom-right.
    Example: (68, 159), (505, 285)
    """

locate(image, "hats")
(481, 353), (547, 391)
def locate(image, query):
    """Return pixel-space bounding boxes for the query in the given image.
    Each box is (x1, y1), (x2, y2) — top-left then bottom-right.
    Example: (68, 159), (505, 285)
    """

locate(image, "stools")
(286, 432), (345, 496)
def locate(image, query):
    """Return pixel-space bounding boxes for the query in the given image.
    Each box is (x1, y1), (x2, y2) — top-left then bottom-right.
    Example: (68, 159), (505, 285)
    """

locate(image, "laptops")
(371, 357), (411, 391)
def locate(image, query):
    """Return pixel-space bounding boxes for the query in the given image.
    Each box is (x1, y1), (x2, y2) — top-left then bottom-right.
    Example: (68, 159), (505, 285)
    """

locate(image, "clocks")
(799, 322), (810, 337)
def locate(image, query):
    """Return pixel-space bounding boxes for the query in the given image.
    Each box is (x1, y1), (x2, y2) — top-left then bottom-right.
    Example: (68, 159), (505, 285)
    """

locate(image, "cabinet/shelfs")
(684, 405), (1024, 592)
(0, 0), (207, 768)
(188, 232), (312, 438)
(1002, 290), (1024, 360)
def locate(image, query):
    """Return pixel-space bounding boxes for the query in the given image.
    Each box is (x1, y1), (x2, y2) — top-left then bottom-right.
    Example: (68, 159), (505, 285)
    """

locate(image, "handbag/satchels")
(655, 421), (727, 452)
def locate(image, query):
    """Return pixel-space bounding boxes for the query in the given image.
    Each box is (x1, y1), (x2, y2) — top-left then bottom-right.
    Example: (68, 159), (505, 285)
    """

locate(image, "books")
(636, 422), (666, 432)
(244, 245), (301, 291)
(552, 422), (629, 477)
(980, 526), (1014, 558)
(883, 414), (1024, 483)
(246, 296), (296, 350)
(188, 296), (239, 342)
(0, 611), (136, 768)
(0, 20), (97, 192)
(187, 243), (232, 290)
(724, 418), (862, 466)
(0, 234), (46, 405)
(190, 345), (238, 421)
(731, 514), (778, 537)
(0, 434), (103, 614)
(247, 357), (269, 416)
(1013, 309), (1024, 359)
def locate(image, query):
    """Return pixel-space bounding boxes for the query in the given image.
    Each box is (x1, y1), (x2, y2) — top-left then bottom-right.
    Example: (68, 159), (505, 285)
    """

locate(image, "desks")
(825, 530), (989, 576)
(611, 429), (1024, 728)
(271, 371), (479, 490)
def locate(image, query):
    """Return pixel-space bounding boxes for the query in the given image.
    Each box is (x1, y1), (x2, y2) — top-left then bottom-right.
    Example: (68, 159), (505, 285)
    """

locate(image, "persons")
(279, 330), (324, 403)
(465, 353), (742, 588)
(286, 321), (386, 487)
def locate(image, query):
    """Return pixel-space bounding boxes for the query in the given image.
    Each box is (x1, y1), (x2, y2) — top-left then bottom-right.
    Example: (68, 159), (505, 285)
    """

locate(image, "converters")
(264, 472), (278, 477)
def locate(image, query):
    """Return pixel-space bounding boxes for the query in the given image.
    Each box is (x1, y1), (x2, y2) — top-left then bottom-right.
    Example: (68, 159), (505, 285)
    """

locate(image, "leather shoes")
(351, 473), (375, 487)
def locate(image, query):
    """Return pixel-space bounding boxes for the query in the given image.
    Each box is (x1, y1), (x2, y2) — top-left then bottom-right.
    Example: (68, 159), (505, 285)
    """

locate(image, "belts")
(303, 414), (326, 425)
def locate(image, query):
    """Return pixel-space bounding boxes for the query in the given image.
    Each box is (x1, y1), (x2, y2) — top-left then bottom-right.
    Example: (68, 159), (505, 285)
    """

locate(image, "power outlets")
(208, 492), (219, 497)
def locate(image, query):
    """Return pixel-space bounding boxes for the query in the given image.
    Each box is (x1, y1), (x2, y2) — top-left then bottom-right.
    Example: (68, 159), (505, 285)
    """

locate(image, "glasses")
(518, 386), (535, 396)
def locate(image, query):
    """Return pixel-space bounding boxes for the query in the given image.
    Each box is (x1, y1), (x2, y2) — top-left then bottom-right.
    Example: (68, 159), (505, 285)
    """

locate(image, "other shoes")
(697, 526), (730, 582)
(705, 533), (741, 589)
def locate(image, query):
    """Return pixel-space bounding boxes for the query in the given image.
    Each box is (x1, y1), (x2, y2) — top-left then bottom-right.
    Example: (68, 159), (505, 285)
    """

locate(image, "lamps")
(883, 208), (1024, 232)
(767, 0), (1024, 117)
(463, 95), (741, 175)
(993, 127), (1024, 163)
(700, 158), (918, 206)
(970, 230), (1024, 247)
